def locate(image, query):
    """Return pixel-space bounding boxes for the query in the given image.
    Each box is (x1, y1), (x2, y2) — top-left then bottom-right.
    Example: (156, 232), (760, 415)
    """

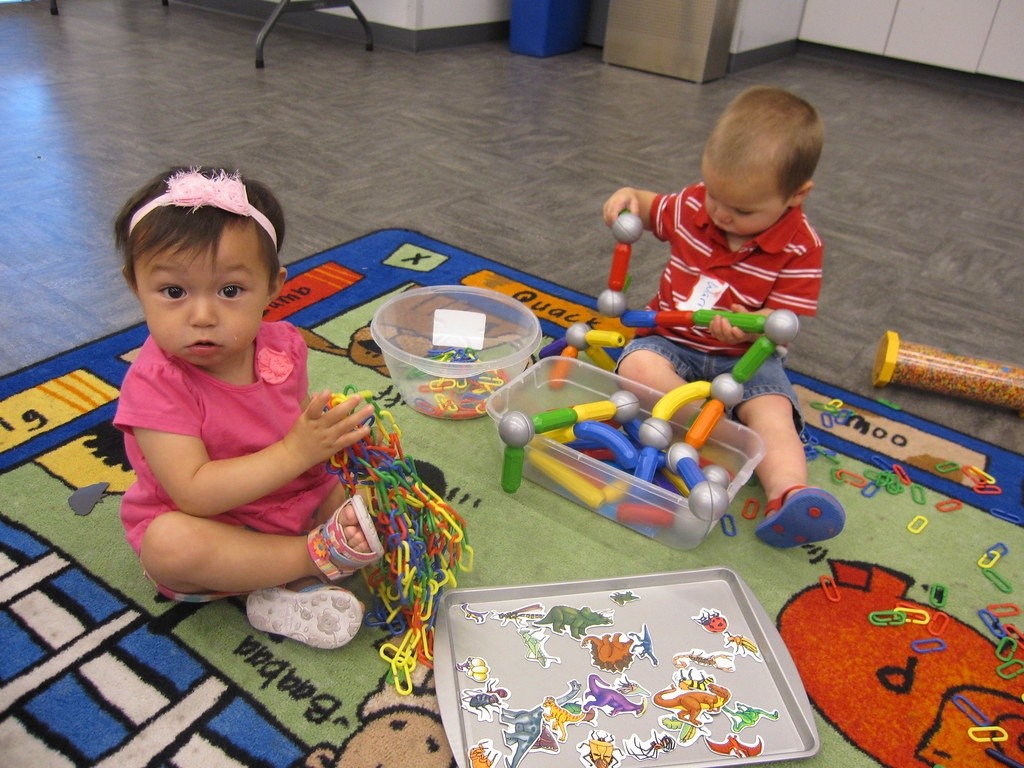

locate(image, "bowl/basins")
(366, 284), (542, 421)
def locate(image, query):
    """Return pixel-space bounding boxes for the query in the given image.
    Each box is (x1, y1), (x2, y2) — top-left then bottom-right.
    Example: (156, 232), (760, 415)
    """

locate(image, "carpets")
(0, 228), (1022, 768)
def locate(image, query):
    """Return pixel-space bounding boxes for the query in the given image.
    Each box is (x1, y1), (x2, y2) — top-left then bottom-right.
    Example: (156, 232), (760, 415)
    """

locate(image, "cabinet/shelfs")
(730, 0), (1023, 82)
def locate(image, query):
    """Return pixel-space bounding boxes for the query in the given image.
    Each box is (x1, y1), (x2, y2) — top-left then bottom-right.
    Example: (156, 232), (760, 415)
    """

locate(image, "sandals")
(305, 493), (386, 585)
(245, 583), (362, 650)
(755, 485), (846, 550)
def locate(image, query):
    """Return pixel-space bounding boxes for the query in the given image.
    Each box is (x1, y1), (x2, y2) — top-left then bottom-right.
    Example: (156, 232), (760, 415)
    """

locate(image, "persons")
(600, 86), (849, 548)
(112, 165), (390, 648)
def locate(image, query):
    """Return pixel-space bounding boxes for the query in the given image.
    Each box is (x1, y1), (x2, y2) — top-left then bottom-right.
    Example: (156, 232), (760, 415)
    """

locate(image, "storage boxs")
(370, 285), (542, 420)
(484, 356), (766, 551)
